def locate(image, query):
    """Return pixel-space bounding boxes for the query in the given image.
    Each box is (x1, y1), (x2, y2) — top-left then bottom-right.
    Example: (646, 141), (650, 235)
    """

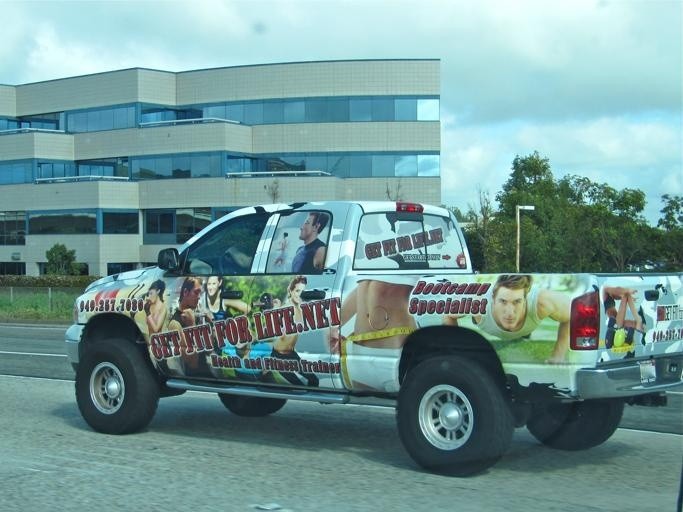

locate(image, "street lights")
(514, 202), (534, 274)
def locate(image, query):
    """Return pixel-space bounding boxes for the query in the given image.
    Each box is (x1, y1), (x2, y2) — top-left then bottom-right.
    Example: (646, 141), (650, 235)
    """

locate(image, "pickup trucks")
(62, 196), (680, 477)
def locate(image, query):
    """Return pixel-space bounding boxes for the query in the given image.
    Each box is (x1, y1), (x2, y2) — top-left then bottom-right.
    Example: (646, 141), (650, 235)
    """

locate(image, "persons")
(224, 247), (252, 268)
(291, 212), (329, 273)
(326, 274), (418, 396)
(443, 275), (571, 364)
(273, 232), (289, 266)
(602, 285), (646, 359)
(144, 274), (319, 387)
